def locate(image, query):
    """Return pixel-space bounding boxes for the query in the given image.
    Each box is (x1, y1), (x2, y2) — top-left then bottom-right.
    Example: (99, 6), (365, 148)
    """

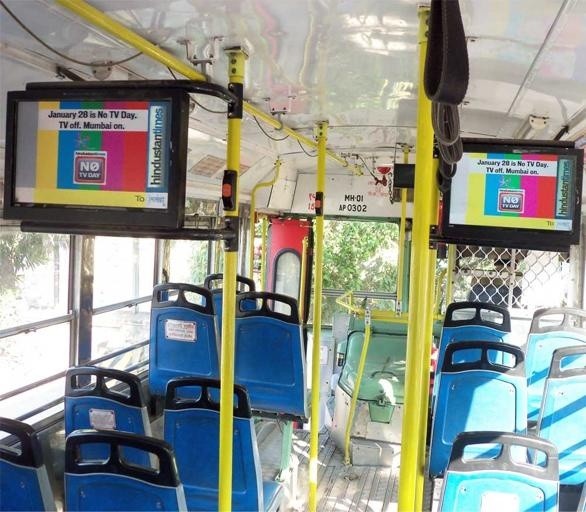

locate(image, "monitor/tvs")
(441, 141), (585, 254)
(2, 90), (191, 231)
(393, 164), (416, 189)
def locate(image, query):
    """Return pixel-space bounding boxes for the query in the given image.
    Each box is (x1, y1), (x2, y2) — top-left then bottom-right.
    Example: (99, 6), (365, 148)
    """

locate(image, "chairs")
(431, 431), (560, 512)
(64, 429), (188, 512)
(0, 417), (57, 512)
(201, 272), (256, 345)
(428, 341), (528, 477)
(64, 366), (160, 474)
(527, 345), (586, 486)
(163, 376), (285, 512)
(431, 301), (511, 415)
(148, 283), (221, 404)
(212, 291), (312, 419)
(523, 307), (586, 423)
